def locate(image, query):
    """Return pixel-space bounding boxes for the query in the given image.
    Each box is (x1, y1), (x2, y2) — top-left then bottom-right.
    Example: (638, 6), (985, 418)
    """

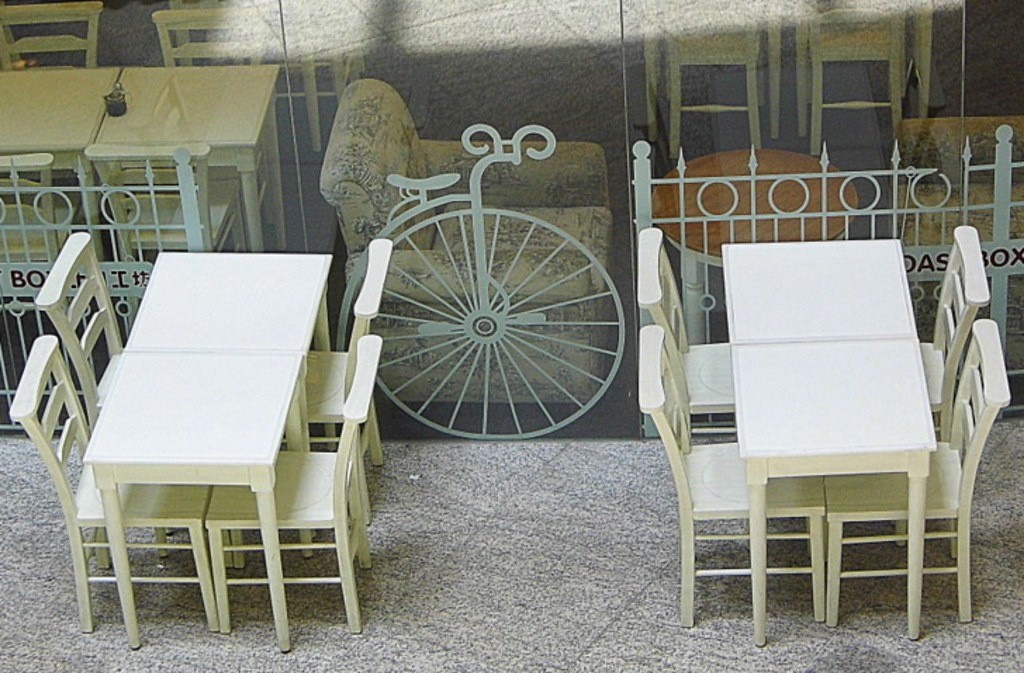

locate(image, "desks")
(84, 252), (333, 655)
(722, 239), (937, 648)
(652, 149), (859, 345)
(0, 65), (285, 252)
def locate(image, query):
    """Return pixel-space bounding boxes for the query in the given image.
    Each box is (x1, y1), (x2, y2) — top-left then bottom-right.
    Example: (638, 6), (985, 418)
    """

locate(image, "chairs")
(826, 226), (1011, 627)
(0, 1), (273, 177)
(638, 227), (826, 623)
(214, 239), (393, 635)
(10, 232), (219, 633)
(0, 142), (246, 333)
(643, 0), (933, 159)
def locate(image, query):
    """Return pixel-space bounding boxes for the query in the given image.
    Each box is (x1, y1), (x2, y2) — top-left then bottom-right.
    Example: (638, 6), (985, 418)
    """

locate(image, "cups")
(104, 90), (133, 117)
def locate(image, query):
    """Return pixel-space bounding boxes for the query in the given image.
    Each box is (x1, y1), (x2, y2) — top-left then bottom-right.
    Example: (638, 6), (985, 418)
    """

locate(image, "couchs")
(893, 116), (1024, 369)
(319, 79), (613, 402)
